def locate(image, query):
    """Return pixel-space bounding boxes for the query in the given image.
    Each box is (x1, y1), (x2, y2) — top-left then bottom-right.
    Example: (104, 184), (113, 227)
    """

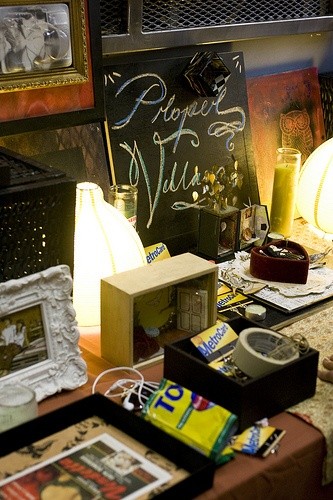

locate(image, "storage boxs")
(250, 240), (310, 284)
(199, 204), (241, 264)
(163, 315), (319, 436)
(101, 252), (219, 376)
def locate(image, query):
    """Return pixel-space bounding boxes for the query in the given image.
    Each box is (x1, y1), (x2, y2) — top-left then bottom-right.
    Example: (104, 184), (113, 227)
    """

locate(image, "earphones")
(122, 393), (134, 411)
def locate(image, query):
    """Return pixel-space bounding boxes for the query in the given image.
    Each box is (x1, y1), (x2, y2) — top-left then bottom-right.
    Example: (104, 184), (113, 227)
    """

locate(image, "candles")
(269, 162), (299, 238)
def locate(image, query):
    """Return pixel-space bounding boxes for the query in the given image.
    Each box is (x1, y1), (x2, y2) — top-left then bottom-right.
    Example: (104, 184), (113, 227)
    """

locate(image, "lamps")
(298, 138), (333, 235)
(72, 180), (148, 326)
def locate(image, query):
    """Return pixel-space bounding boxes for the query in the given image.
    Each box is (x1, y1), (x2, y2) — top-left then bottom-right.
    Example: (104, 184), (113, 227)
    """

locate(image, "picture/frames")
(0, 0), (107, 137)
(0, 264), (88, 408)
(0, 392), (217, 500)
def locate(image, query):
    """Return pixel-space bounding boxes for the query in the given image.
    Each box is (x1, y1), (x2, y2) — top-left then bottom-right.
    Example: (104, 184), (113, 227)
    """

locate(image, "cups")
(0, 384), (38, 433)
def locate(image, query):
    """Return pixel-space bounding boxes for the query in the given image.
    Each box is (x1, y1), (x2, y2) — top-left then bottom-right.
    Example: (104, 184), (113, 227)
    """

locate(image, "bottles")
(108, 184), (138, 231)
(268, 148), (302, 238)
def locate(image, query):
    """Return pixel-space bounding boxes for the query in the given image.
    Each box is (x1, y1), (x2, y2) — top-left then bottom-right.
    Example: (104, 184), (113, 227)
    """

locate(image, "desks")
(0, 220), (333, 500)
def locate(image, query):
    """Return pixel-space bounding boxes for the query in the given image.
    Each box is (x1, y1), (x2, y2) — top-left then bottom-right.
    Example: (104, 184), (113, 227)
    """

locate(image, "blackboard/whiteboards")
(100, 50), (262, 256)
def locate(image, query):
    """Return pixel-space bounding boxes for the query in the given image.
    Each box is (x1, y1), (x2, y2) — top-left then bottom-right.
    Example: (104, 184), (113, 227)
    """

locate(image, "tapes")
(234, 327), (300, 379)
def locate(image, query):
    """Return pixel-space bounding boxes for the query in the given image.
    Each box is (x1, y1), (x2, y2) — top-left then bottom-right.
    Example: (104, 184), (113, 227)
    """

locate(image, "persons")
(0, 316), (30, 378)
(317, 354), (333, 383)
